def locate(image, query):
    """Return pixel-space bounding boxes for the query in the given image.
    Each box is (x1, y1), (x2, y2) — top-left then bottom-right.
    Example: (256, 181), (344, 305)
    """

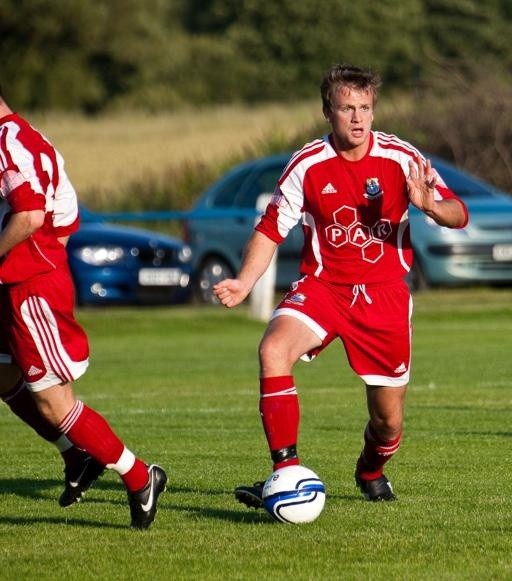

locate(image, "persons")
(211, 66), (469, 509)
(0, 100), (166, 529)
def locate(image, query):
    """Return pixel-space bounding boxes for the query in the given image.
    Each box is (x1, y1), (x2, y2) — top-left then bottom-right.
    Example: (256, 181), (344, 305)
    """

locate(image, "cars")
(181, 153), (512, 305)
(65, 203), (195, 306)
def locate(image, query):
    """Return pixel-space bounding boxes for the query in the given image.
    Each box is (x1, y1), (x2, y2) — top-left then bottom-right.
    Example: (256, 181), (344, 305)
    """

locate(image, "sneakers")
(59, 451), (107, 508)
(127, 462), (169, 530)
(354, 469), (397, 502)
(234, 480), (265, 510)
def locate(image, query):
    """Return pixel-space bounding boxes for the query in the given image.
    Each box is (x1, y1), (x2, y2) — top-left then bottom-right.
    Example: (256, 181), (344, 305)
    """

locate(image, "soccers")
(263, 464), (325, 523)
